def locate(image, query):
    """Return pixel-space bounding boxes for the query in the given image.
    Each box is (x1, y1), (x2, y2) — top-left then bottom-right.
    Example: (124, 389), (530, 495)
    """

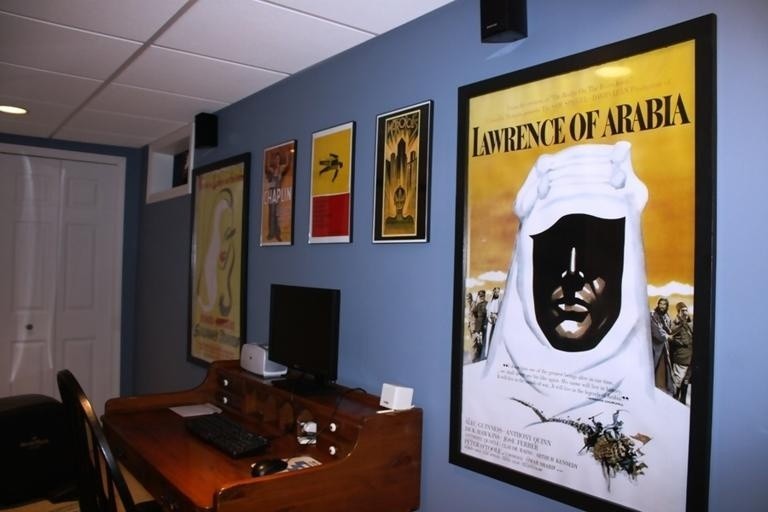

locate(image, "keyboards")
(182, 412), (268, 458)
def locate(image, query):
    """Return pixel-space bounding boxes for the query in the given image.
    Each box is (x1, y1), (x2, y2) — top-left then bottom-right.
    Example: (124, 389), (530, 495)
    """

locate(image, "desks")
(100, 360), (422, 512)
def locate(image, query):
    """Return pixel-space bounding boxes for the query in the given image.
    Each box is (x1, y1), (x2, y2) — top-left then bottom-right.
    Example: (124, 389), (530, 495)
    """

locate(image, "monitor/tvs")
(268, 284), (341, 398)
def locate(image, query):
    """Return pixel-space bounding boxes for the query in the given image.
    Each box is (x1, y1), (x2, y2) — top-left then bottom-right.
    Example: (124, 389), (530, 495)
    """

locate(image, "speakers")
(195, 112), (218, 148)
(376, 383), (415, 413)
(480, 0), (528, 43)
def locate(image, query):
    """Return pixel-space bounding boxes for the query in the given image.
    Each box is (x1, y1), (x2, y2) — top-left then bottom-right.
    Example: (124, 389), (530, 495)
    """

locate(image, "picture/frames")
(187, 151), (251, 368)
(307, 120), (356, 245)
(259, 139), (299, 247)
(448, 11), (716, 511)
(371, 99), (434, 244)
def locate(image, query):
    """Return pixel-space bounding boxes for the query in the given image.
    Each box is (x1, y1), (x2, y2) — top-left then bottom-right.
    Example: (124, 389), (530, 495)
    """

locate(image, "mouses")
(250, 459), (287, 476)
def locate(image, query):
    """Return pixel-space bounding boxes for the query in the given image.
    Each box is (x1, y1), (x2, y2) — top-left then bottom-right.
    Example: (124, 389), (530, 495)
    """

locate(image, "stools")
(0, 394), (62, 509)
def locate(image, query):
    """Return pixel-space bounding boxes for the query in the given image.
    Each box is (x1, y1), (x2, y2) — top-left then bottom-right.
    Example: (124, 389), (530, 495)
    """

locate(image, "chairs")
(57, 370), (164, 512)
